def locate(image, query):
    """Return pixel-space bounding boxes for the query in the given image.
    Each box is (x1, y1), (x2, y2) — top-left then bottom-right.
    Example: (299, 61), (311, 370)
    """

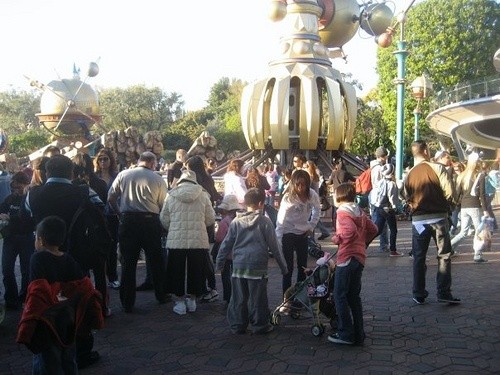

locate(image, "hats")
(175, 170), (196, 186)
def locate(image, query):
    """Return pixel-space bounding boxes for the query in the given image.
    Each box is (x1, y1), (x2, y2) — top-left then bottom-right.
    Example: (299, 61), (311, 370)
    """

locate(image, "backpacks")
(66, 184), (112, 270)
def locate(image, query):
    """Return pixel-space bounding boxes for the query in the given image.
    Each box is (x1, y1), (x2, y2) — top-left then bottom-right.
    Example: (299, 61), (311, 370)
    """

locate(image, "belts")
(121, 212), (157, 218)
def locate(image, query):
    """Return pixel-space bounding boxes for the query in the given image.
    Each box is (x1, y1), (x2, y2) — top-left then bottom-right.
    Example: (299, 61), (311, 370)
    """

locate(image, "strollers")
(270, 248), (337, 337)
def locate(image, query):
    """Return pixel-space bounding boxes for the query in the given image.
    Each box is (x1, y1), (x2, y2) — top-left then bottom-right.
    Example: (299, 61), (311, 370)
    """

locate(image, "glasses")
(98, 157), (110, 161)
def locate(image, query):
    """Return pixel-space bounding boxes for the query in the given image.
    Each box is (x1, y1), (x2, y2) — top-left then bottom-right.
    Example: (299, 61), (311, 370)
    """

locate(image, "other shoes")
(437, 297), (462, 303)
(412, 297), (426, 304)
(107, 281), (120, 289)
(187, 298), (197, 312)
(173, 302), (186, 315)
(474, 257), (488, 263)
(390, 251), (404, 256)
(77, 350), (99, 368)
(328, 332), (364, 344)
(199, 288), (219, 302)
(318, 235), (330, 240)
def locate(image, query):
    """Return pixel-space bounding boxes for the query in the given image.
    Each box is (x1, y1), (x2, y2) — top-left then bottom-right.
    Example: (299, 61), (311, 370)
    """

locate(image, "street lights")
(376, 0), (419, 182)
(411, 74), (433, 142)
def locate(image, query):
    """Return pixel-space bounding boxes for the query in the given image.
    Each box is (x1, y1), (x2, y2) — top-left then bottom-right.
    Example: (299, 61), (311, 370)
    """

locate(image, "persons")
(431, 148), (498, 263)
(18, 215), (104, 375)
(0, 145), (331, 332)
(369, 146), (404, 256)
(327, 159), (356, 232)
(214, 194), (241, 306)
(330, 184), (379, 345)
(403, 139), (460, 305)
(275, 169), (321, 301)
(293, 253), (335, 296)
(216, 188), (291, 336)
